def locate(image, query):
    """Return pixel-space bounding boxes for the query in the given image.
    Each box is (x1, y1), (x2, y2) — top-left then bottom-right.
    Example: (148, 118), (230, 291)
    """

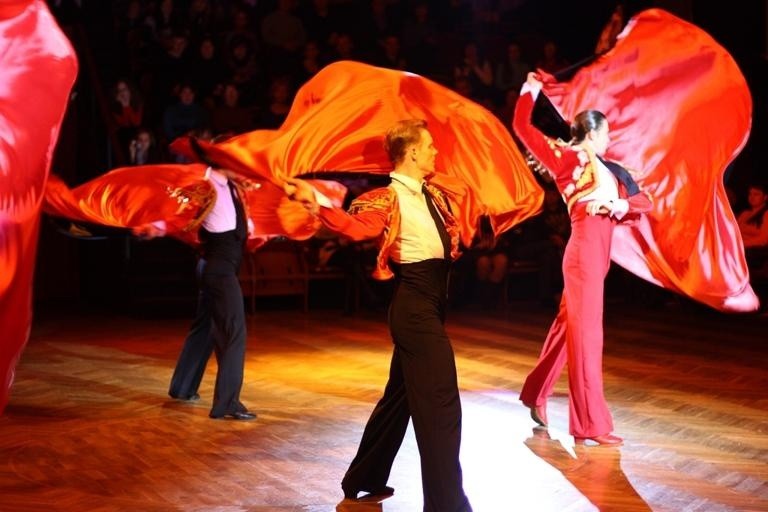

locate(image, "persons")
(513, 70), (645, 449)
(286, 116), (471, 511)
(135, 127), (256, 422)
(57, 2), (767, 281)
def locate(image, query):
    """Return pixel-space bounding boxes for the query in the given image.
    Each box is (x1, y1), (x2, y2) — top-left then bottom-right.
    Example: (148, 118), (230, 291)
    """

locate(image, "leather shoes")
(170, 393), (199, 400)
(210, 410), (257, 419)
(573, 435), (623, 445)
(522, 398), (547, 426)
(342, 486), (394, 499)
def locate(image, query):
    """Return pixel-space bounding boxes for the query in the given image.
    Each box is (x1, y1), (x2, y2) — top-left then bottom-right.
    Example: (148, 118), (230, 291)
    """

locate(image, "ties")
(227, 180), (250, 257)
(595, 154), (641, 198)
(422, 186), (457, 279)
